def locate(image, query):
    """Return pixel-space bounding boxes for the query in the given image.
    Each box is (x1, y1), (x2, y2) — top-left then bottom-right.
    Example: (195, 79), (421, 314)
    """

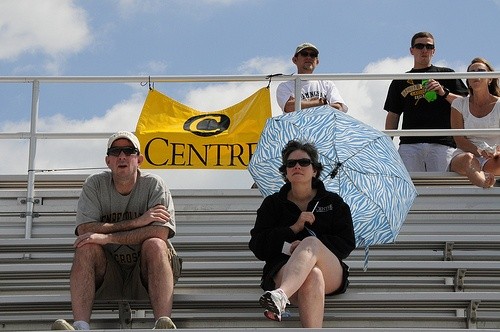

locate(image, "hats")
(108, 131), (140, 153)
(296, 43), (319, 53)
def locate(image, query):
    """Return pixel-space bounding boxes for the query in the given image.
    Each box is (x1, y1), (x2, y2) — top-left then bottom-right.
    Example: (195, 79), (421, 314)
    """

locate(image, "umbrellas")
(249, 104), (419, 273)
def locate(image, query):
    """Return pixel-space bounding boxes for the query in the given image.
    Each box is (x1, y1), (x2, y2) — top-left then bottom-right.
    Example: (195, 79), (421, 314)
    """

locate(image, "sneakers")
(259, 291), (287, 322)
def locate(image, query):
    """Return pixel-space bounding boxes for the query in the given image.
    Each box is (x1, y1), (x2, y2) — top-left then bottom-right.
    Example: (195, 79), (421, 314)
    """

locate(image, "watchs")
(438, 87), (449, 99)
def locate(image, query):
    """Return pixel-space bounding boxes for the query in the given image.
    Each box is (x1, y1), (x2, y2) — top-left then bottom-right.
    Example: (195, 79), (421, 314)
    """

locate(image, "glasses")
(298, 51), (318, 57)
(286, 158), (313, 168)
(413, 44), (434, 50)
(107, 146), (139, 156)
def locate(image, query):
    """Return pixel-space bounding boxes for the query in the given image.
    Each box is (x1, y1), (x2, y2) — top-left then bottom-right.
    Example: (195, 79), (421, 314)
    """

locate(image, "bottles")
(422, 72), (436, 102)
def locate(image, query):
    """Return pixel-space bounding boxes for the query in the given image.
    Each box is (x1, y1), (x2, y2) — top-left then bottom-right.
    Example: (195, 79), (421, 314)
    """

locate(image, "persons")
(50, 131), (183, 330)
(248, 141), (356, 328)
(383, 33), (468, 172)
(446, 57), (500, 190)
(276, 42), (348, 117)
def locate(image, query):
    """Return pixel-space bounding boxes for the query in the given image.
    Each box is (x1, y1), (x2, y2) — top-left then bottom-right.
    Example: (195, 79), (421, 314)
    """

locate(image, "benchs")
(0, 177), (500, 332)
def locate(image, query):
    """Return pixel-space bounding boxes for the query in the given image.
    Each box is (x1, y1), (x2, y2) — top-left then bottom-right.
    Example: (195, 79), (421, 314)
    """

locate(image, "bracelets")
(319, 96), (327, 105)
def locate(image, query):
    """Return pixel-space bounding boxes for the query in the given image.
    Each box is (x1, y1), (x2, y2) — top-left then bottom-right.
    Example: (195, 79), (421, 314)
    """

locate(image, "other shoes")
(51, 319), (87, 330)
(153, 317), (176, 329)
